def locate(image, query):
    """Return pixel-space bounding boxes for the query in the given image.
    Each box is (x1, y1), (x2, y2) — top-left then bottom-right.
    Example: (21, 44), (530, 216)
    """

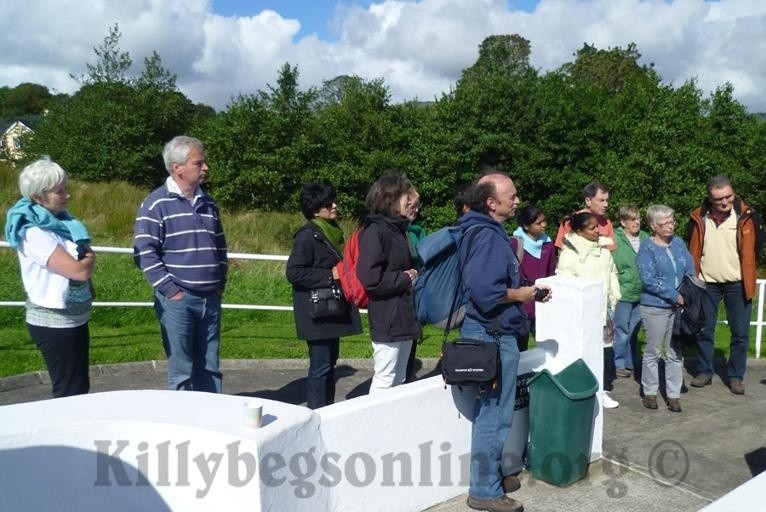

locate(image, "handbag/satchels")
(307, 287), (350, 320)
(440, 338), (501, 392)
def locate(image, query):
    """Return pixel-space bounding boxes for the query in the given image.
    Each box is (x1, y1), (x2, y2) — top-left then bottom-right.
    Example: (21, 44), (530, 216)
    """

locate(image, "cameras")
(520, 277), (549, 302)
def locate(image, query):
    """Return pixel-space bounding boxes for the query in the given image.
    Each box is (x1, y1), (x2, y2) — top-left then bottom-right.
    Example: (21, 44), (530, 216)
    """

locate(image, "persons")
(3, 158), (96, 397)
(353, 170), (421, 393)
(285, 177), (366, 409)
(453, 172), (553, 511)
(405, 184), (430, 382)
(132, 135), (228, 395)
(447, 174), (766, 416)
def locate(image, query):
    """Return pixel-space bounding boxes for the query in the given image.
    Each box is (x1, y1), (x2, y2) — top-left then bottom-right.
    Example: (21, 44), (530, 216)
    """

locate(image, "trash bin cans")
(526, 358), (599, 488)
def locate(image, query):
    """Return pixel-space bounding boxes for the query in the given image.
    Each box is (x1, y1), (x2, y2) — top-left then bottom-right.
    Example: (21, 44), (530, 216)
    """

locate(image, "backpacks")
(413, 223), (507, 331)
(336, 219), (391, 309)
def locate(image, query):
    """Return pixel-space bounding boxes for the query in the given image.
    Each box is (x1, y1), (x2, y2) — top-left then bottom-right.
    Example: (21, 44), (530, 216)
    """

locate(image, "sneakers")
(615, 368), (631, 378)
(690, 373), (713, 388)
(643, 395), (658, 409)
(726, 378), (746, 395)
(666, 397), (681, 413)
(467, 494), (524, 512)
(602, 390), (618, 408)
(501, 475), (521, 491)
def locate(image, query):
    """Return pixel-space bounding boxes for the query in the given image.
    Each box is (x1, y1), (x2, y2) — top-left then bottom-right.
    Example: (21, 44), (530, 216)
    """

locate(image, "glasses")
(656, 220), (677, 228)
